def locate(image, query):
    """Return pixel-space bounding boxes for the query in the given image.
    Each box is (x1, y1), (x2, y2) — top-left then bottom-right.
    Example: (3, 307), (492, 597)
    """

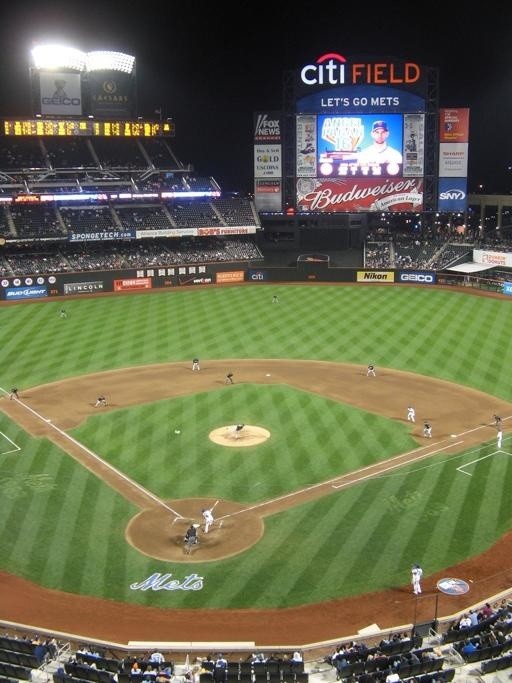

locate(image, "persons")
(492, 414), (501, 431)
(422, 421), (432, 438)
(192, 358), (200, 371)
(272, 295), (279, 302)
(23, 597), (509, 683)
(366, 363), (376, 376)
(360, 119), (402, 175)
(202, 509), (223, 533)
(410, 564), (423, 595)
(407, 406), (416, 423)
(59, 305), (67, 317)
(224, 373), (234, 384)
(184, 524), (199, 544)
(364, 227), (512, 293)
(496, 428), (503, 447)
(94, 395), (107, 407)
(10, 386), (19, 400)
(227, 424), (246, 440)
(1, 135), (261, 278)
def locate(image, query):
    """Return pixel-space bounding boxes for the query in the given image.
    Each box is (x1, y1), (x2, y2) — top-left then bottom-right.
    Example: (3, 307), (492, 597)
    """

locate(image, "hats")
(372, 121), (388, 131)
